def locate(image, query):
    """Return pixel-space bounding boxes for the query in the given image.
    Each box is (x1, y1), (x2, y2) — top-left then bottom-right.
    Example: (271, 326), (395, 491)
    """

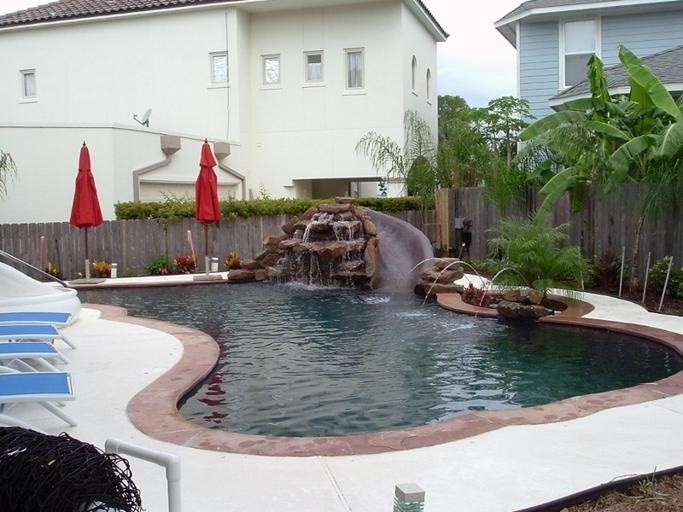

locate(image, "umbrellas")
(69, 145), (104, 283)
(195, 143), (222, 280)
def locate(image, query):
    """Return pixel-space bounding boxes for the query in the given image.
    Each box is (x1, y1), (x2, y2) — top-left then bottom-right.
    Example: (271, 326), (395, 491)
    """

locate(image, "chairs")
(0, 310), (77, 427)
